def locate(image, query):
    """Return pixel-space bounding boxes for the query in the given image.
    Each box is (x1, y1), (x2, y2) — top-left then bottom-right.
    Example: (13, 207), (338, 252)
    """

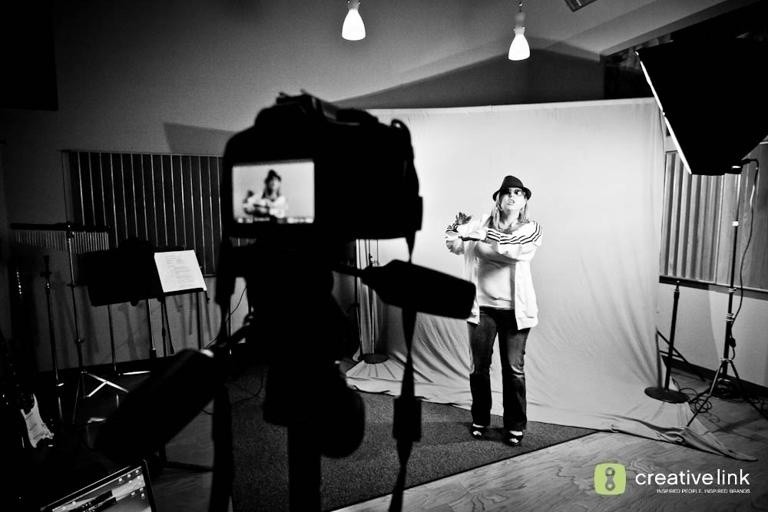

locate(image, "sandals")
(471, 423), (486, 439)
(505, 430), (524, 446)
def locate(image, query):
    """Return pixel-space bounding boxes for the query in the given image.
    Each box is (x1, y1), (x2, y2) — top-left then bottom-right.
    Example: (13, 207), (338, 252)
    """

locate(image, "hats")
(265, 170), (282, 183)
(493, 176), (531, 202)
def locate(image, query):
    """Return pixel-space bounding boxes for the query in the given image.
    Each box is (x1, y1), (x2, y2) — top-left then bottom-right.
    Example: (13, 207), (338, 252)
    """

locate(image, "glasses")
(502, 188), (522, 195)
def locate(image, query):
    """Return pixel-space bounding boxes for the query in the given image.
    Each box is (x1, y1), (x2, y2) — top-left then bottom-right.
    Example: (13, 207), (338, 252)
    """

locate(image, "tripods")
(684, 172), (768, 430)
(51, 237), (130, 426)
(85, 304), (152, 411)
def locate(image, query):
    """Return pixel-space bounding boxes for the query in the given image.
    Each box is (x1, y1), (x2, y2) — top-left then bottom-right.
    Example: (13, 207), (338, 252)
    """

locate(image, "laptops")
(40, 459), (157, 512)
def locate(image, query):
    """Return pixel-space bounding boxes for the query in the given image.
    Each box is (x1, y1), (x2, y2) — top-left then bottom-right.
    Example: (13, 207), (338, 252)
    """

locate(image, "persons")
(443, 174), (545, 446)
(240, 167), (291, 223)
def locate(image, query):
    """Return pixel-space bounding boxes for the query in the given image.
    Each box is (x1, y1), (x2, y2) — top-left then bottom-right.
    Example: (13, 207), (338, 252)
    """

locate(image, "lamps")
(507, 0), (529, 60)
(340, 0), (365, 40)
